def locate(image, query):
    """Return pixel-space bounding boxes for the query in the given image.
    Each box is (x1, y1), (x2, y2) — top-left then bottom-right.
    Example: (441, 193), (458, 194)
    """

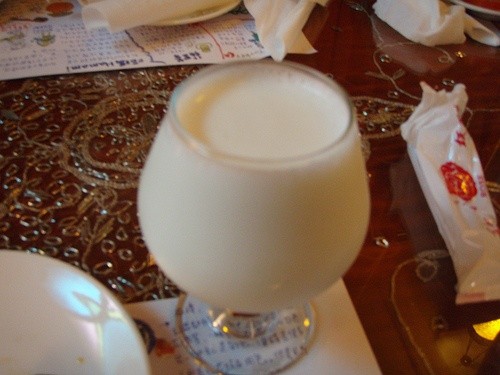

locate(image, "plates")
(0, 249), (151, 375)
(451, 0), (500, 22)
(77, 0), (241, 26)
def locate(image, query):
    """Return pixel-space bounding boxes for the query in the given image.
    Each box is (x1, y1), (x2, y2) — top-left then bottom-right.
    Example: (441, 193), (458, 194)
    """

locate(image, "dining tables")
(0, 0), (500, 375)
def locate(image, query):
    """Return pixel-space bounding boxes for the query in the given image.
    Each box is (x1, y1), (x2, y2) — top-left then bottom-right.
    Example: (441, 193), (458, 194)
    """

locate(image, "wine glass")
(137, 59), (369, 375)
